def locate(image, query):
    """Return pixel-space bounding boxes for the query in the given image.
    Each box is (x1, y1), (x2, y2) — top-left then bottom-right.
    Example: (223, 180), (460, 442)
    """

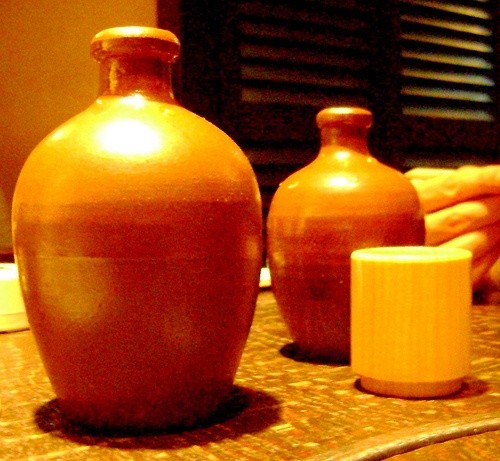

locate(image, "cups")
(350, 246), (472, 400)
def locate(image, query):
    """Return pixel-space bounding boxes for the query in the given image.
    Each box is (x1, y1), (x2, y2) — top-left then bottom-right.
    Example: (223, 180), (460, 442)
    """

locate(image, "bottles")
(266, 107), (427, 360)
(11, 26), (263, 433)
